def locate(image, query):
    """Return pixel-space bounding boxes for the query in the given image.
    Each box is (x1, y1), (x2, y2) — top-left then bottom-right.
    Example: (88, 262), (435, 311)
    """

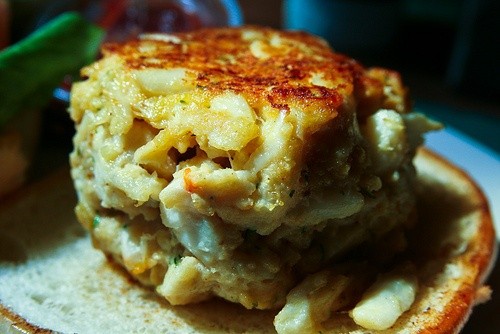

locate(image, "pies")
(0, 146), (499, 333)
(66, 26), (446, 334)
